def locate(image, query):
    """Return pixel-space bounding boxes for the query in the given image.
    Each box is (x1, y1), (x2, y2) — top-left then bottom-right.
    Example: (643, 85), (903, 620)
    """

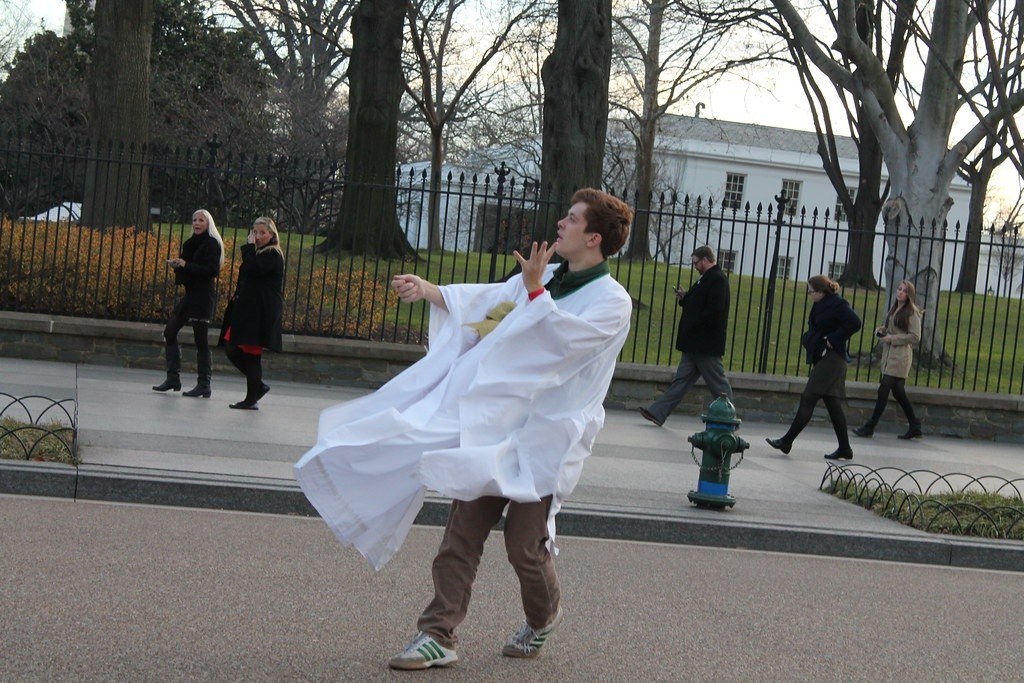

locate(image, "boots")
(153, 347), (181, 391)
(898, 417), (922, 439)
(765, 394), (815, 454)
(182, 351), (213, 398)
(852, 417), (877, 437)
(824, 408), (853, 459)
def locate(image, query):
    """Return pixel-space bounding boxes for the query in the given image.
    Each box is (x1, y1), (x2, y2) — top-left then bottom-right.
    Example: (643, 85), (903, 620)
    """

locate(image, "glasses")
(694, 257), (702, 264)
(808, 290), (816, 293)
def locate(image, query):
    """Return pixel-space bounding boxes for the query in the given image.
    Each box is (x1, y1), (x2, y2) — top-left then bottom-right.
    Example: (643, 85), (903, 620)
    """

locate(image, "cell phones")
(877, 332), (885, 337)
(673, 286), (678, 293)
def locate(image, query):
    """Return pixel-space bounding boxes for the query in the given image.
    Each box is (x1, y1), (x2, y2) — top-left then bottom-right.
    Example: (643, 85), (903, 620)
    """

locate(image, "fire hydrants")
(686, 393), (750, 510)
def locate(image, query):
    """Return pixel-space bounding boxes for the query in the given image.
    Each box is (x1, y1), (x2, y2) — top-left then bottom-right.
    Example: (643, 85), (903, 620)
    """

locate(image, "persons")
(852, 281), (923, 439)
(217, 217), (285, 410)
(153, 210), (224, 398)
(640, 246), (739, 429)
(295, 190), (632, 669)
(765, 275), (862, 459)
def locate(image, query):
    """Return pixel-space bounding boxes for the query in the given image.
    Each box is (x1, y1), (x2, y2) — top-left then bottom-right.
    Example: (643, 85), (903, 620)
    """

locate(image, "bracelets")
(528, 288), (544, 300)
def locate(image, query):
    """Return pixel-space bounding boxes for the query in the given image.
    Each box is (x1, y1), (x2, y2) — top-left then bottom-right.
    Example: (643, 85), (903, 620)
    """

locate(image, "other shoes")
(256, 383), (268, 400)
(639, 407), (662, 426)
(229, 400), (258, 409)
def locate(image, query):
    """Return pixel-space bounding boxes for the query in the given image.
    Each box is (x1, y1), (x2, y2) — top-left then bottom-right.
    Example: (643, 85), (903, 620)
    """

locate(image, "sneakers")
(388, 630), (458, 668)
(502, 606), (564, 657)
